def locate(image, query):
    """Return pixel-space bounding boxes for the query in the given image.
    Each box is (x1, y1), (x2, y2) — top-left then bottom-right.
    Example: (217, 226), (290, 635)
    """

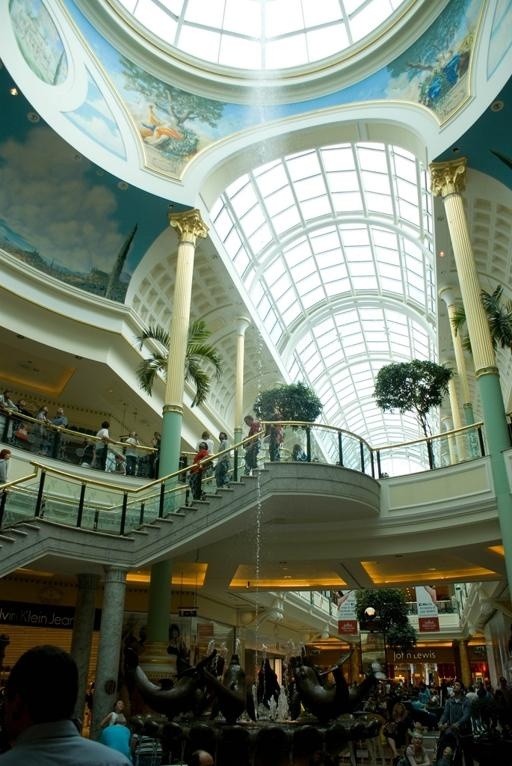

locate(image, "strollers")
(431, 729), (465, 766)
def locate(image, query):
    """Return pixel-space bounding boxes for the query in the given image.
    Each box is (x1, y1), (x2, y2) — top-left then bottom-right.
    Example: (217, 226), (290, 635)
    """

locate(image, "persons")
(382, 673), (512, 766)
(179, 453), (187, 483)
(196, 432), (214, 455)
(269, 424), (284, 463)
(243, 415), (262, 476)
(0, 388), (160, 529)
(187, 749), (214, 765)
(1, 643), (133, 765)
(189, 441), (210, 501)
(146, 103), (159, 129)
(214, 432), (230, 495)
(123, 617), (143, 659)
(167, 623), (191, 675)
(293, 444), (308, 462)
